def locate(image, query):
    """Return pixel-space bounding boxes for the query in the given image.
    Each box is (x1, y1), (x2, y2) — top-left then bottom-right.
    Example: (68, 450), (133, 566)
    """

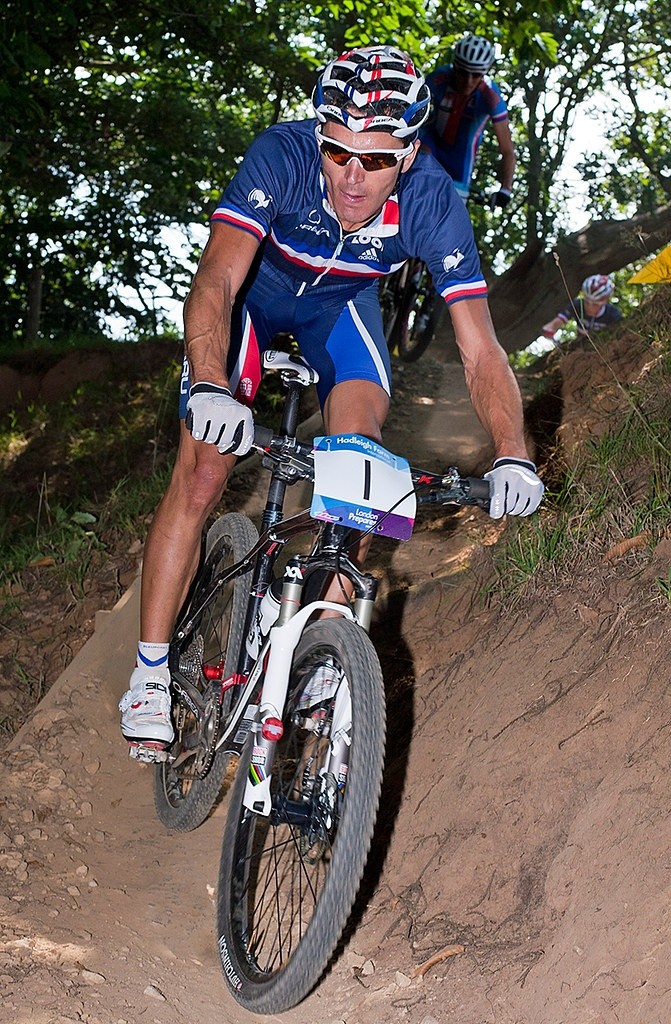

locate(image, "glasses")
(456, 66), (483, 80)
(310, 120), (415, 172)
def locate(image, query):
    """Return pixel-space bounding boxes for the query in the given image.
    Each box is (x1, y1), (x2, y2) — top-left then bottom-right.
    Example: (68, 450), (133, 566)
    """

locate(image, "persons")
(378, 32), (516, 342)
(540, 272), (628, 370)
(117, 44), (547, 767)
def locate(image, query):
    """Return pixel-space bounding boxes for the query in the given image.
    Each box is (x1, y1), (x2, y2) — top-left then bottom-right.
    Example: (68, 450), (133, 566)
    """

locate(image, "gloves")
(488, 186), (512, 213)
(186, 379), (256, 457)
(482, 456), (546, 518)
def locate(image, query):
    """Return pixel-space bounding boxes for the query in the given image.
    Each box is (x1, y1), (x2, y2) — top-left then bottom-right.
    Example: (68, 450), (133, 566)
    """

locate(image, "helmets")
(453, 32), (498, 75)
(306, 42), (432, 139)
(578, 274), (617, 305)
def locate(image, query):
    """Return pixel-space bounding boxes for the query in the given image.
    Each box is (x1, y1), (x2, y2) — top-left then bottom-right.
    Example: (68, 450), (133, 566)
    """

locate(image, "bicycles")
(128, 348), (515, 1015)
(374, 189), (499, 362)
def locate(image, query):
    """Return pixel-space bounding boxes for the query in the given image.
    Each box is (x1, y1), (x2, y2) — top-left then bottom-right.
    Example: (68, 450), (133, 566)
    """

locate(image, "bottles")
(245, 576), (283, 662)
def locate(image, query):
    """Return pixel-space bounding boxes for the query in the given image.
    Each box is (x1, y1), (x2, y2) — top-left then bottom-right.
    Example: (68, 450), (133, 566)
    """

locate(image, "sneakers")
(294, 650), (343, 730)
(116, 673), (176, 747)
